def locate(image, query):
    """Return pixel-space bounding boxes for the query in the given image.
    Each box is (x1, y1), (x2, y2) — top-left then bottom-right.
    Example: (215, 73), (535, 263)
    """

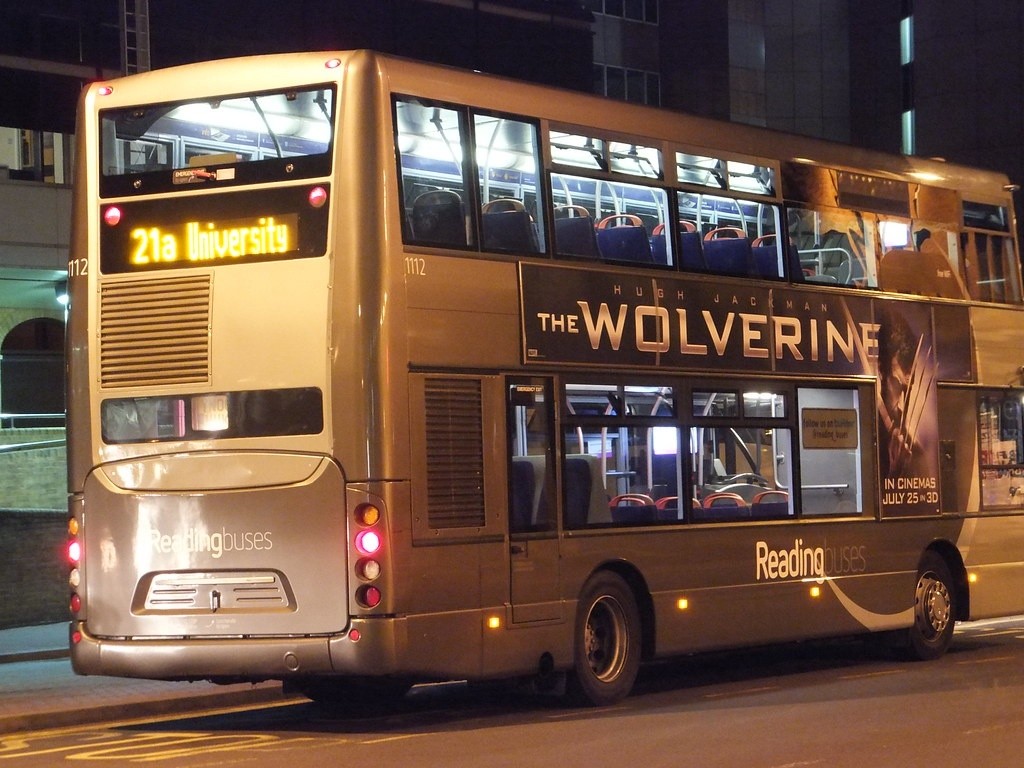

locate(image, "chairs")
(808, 231), (867, 287)
(408, 190), (806, 282)
(612, 484), (791, 525)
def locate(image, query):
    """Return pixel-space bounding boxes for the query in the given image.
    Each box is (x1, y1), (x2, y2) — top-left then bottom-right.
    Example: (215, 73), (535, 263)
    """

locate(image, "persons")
(877, 296), (930, 518)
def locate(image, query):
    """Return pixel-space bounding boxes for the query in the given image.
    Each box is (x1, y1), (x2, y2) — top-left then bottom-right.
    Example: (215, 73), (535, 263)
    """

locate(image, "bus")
(61, 46), (1024, 713)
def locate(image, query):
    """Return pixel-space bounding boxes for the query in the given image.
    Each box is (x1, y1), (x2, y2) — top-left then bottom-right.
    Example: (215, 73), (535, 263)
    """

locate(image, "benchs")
(511, 455), (601, 527)
